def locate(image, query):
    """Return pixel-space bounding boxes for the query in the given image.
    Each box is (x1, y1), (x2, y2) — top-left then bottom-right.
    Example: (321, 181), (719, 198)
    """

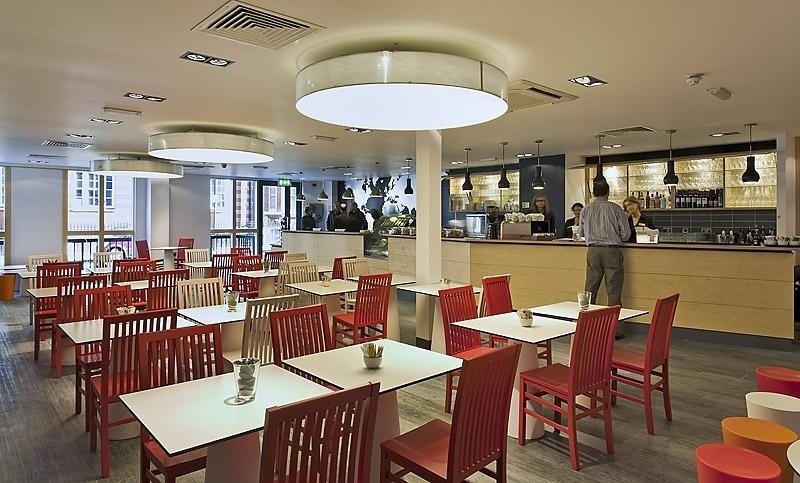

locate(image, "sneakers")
(615, 333), (624, 340)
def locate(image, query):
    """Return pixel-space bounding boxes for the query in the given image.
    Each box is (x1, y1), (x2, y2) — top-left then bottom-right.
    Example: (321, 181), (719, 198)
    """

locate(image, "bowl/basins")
(363, 356), (383, 369)
(440, 278), (451, 285)
(520, 317), (533, 327)
(763, 235), (799, 245)
(117, 305), (136, 315)
(322, 281), (330, 287)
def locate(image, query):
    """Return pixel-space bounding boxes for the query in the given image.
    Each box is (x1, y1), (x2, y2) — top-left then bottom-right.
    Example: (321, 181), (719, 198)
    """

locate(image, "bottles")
(720, 229), (736, 243)
(738, 224), (775, 245)
(675, 188), (724, 208)
(630, 188), (666, 209)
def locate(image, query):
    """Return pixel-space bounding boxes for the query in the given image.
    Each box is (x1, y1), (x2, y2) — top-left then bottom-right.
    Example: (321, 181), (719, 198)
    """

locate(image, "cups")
(233, 356), (261, 401)
(570, 226), (581, 239)
(226, 290), (239, 314)
(576, 291), (592, 311)
(262, 259), (271, 274)
(449, 192), (484, 211)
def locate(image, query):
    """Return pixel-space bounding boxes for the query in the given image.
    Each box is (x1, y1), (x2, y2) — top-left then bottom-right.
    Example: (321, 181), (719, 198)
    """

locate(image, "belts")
(588, 243), (619, 249)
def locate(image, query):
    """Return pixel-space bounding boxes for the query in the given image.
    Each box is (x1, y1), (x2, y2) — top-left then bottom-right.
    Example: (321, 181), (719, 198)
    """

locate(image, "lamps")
(318, 168), (329, 200)
(90, 159), (183, 178)
(296, 52), (509, 132)
(593, 135), (606, 183)
(742, 123), (760, 182)
(404, 158), (413, 194)
(498, 142), (510, 189)
(462, 148), (473, 190)
(296, 171), (305, 201)
(532, 139), (546, 187)
(147, 133), (275, 164)
(664, 129), (679, 185)
(342, 165), (354, 199)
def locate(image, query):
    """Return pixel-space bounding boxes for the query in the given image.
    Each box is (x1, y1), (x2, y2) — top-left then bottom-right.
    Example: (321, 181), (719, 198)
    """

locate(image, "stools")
(696, 365), (800, 483)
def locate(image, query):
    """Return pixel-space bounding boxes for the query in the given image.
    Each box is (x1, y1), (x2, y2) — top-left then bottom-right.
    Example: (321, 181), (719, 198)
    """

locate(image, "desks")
(0, 264), (91, 296)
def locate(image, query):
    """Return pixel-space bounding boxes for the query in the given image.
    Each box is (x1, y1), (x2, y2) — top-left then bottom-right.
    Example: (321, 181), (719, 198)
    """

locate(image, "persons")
(623, 195), (655, 243)
(326, 200), (368, 232)
(302, 206), (316, 230)
(529, 193), (555, 233)
(562, 202), (583, 238)
(579, 180), (631, 341)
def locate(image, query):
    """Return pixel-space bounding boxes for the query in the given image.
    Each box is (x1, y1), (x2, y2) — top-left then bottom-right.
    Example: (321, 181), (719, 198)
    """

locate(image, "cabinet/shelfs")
(448, 170), (519, 212)
(588, 151), (777, 211)
